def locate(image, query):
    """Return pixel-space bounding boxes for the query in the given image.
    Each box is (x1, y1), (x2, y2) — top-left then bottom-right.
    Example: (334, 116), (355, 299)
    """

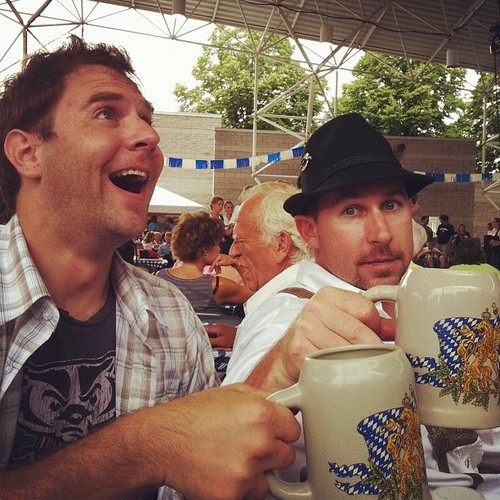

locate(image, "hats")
(283, 112), (436, 216)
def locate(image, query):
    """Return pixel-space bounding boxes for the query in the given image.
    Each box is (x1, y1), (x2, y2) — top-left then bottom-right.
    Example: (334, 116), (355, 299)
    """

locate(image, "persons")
(218, 111), (500, 500)
(1, 34), (301, 500)
(118, 181), (500, 348)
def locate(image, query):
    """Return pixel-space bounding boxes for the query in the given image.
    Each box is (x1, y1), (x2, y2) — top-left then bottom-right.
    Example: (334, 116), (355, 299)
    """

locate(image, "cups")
(360, 257), (499, 431)
(262, 342), (430, 499)
